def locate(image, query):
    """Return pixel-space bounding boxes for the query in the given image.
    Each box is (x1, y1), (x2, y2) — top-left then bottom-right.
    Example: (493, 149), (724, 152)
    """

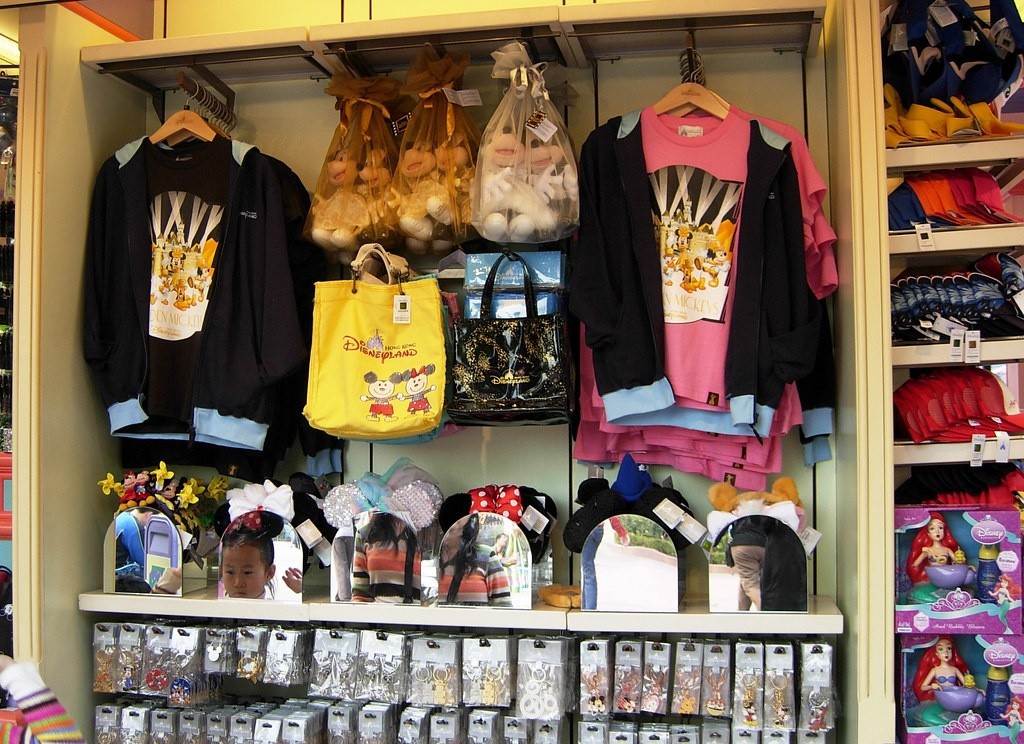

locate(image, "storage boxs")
(896, 633), (1024, 744)
(894, 505), (1022, 634)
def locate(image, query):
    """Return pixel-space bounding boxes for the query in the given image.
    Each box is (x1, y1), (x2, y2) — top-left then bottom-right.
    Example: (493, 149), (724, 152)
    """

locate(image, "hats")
(887, 166), (1024, 445)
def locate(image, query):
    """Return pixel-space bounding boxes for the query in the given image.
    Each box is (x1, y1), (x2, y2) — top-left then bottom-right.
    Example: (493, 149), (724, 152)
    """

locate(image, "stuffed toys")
(306, 121), (580, 263)
(116, 470), (187, 511)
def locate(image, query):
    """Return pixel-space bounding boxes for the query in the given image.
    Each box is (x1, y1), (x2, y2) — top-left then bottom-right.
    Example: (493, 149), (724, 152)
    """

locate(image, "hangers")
(149, 78), (236, 147)
(652, 49), (730, 120)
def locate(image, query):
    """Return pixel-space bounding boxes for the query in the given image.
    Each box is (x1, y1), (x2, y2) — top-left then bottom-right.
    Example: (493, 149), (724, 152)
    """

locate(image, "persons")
(907, 512), (977, 607)
(113, 508), (182, 594)
(329, 509), (422, 605)
(716, 515), (808, 613)
(911, 634), (987, 729)
(438, 514), (530, 607)
(0, 655), (88, 744)
(581, 517), (631, 610)
(219, 510), (303, 602)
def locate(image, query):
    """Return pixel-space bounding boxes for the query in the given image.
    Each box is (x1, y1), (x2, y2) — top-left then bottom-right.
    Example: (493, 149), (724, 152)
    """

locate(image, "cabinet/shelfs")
(871, 0), (1024, 468)
(0, 0), (875, 744)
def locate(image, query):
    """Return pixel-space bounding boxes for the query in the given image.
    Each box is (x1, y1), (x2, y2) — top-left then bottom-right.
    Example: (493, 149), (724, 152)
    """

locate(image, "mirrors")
(218, 510), (303, 603)
(436, 512), (553, 609)
(709, 515), (807, 612)
(330, 510), (421, 606)
(103, 506), (207, 598)
(580, 514), (686, 612)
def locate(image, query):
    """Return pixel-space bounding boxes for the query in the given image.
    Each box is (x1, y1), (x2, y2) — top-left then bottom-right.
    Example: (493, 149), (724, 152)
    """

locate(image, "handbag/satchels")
(445, 252), (570, 425)
(303, 242), (447, 441)
